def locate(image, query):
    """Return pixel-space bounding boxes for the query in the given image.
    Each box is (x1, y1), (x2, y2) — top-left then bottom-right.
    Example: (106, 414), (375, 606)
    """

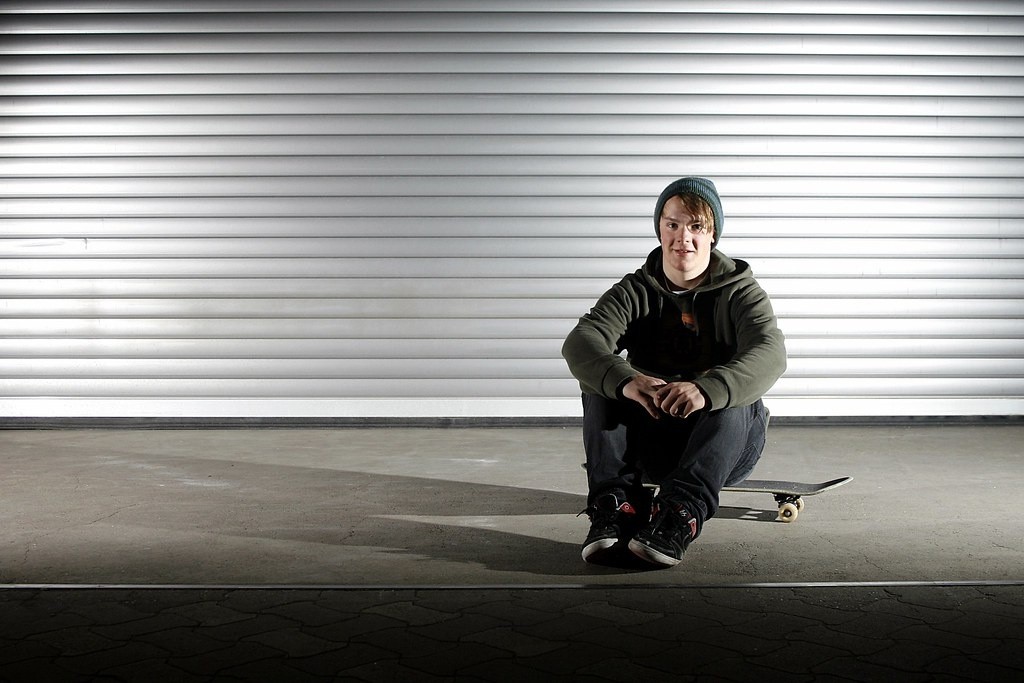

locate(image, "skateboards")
(583, 463), (854, 522)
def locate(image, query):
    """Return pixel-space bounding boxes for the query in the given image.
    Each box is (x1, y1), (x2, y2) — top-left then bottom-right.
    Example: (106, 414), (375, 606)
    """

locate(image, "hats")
(654, 177), (724, 251)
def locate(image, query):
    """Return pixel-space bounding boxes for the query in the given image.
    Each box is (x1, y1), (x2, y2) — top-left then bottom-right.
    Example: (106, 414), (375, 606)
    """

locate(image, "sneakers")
(576, 489), (696, 567)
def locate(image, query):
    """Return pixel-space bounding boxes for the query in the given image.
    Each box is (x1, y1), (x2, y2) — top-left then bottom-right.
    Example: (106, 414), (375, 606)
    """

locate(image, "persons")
(561, 177), (787, 565)
